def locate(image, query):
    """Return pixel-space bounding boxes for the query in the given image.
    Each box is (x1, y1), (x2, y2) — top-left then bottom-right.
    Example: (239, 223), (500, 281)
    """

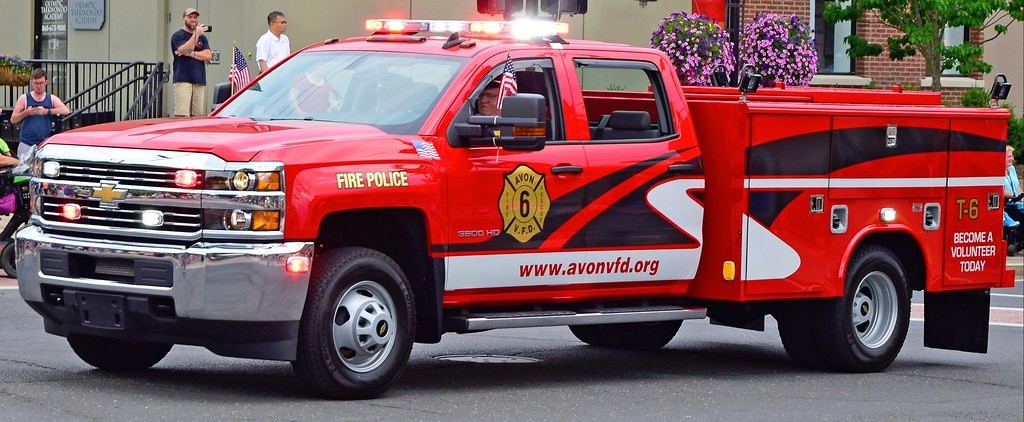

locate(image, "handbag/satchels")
(0, 170), (15, 199)
(0, 192), (18, 214)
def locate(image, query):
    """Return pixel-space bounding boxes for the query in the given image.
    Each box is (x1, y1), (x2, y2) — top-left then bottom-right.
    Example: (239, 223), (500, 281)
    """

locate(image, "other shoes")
(1003, 212), (1020, 227)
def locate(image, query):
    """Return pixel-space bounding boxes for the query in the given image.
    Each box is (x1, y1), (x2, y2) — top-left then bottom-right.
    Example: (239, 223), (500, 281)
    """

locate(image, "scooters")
(0, 142), (36, 279)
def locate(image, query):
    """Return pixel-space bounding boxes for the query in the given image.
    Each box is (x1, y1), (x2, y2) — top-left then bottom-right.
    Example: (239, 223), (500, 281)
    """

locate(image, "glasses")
(275, 20), (287, 25)
(33, 81), (45, 87)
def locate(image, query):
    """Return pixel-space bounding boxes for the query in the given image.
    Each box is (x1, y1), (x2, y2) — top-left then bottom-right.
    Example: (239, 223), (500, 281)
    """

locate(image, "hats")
(183, 8), (199, 18)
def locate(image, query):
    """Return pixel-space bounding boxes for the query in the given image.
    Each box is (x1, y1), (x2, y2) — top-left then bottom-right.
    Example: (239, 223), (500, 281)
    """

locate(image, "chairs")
(594, 110), (656, 139)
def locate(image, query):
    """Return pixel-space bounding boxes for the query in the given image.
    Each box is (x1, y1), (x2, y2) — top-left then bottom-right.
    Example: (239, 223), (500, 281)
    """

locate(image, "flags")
(497, 53), (517, 110)
(228, 46), (250, 92)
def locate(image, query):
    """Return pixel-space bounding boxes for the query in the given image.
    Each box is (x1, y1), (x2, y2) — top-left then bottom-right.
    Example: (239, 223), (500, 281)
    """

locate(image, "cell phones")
(202, 25), (213, 32)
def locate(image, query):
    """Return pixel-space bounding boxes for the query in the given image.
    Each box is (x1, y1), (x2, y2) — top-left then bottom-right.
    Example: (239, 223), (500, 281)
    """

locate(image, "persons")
(379, 74), (413, 112)
(256, 11), (291, 116)
(290, 63), (335, 116)
(171, 9), (212, 117)
(477, 75), (551, 139)
(1003, 146), (1024, 256)
(0, 106), (20, 167)
(11, 69), (69, 212)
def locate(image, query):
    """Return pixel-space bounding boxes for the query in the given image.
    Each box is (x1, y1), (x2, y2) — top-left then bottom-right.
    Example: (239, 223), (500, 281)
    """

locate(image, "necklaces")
(1006, 169), (1014, 193)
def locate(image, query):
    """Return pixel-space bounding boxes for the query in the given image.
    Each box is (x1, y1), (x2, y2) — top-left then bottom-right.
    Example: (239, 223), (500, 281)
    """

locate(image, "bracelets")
(48, 108), (51, 116)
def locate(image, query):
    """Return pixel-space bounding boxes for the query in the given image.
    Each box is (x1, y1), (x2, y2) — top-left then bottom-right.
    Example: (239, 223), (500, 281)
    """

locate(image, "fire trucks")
(14, 20), (1016, 401)
